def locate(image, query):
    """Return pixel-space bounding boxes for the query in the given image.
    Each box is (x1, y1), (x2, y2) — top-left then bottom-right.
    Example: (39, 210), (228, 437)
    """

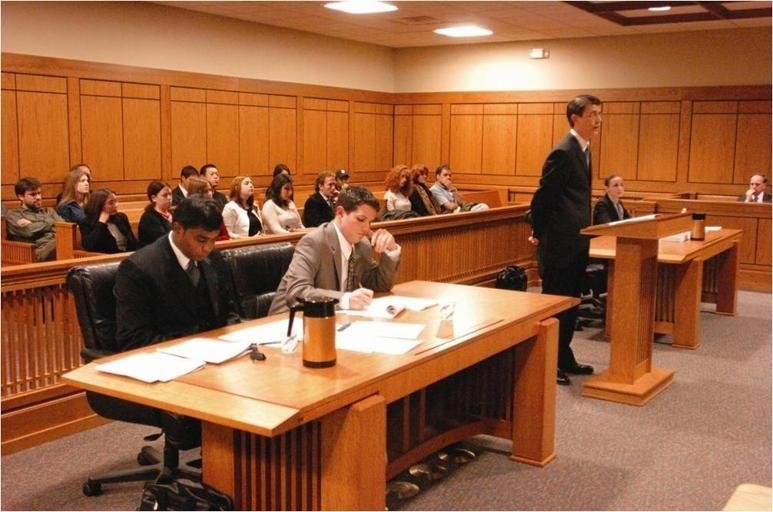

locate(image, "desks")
(588, 227), (743, 350)
(60, 280), (581, 511)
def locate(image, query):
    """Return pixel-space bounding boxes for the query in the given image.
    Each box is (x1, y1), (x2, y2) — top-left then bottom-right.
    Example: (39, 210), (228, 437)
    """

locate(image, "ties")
(348, 248), (356, 290)
(187, 260), (200, 287)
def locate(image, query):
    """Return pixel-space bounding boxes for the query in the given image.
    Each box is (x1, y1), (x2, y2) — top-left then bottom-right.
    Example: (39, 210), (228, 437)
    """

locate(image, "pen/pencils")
(358, 281), (363, 288)
(338, 323), (351, 331)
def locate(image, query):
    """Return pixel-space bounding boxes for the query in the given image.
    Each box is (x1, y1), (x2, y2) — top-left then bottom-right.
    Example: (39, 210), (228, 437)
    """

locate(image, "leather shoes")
(569, 361), (593, 374)
(557, 371), (569, 385)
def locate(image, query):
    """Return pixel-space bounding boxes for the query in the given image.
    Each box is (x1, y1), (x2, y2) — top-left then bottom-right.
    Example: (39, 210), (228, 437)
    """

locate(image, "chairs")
(525, 209), (607, 331)
(221, 241), (295, 322)
(64, 249), (239, 511)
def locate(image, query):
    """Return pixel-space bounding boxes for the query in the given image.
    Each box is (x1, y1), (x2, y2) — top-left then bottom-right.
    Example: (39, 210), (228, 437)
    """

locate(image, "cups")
(436, 320), (455, 340)
(281, 328), (299, 355)
(439, 298), (455, 320)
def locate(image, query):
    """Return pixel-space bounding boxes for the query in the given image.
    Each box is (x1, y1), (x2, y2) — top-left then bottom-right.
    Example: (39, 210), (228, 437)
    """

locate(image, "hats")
(336, 169), (349, 178)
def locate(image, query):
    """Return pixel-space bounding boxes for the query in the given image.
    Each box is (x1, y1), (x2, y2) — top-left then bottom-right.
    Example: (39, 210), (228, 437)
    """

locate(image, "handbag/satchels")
(497, 265), (527, 291)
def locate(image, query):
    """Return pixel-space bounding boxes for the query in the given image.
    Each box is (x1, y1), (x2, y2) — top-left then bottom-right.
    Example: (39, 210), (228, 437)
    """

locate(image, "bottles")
(690, 213), (706, 241)
(680, 208), (688, 241)
(287, 296), (340, 368)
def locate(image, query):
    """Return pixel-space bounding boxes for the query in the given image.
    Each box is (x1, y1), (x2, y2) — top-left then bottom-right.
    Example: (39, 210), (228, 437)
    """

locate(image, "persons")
(6, 162), (137, 260)
(260, 163), (353, 235)
(112, 197), (243, 351)
(267, 186), (401, 310)
(593, 175), (632, 225)
(528, 94), (594, 386)
(140, 165), (265, 246)
(736, 175), (772, 203)
(382, 164), (487, 220)
